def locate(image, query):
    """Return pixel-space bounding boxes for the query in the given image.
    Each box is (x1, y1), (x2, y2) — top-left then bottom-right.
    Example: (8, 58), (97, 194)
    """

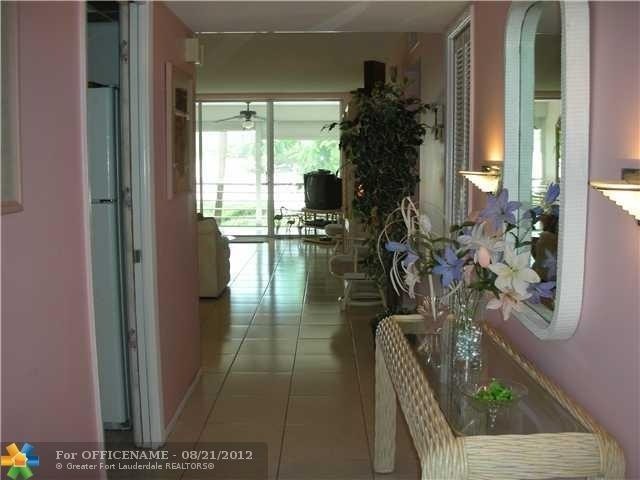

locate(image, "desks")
(370, 311), (629, 480)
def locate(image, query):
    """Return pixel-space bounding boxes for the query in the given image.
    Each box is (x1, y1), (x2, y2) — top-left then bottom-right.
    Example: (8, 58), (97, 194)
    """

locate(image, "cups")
(621, 168), (640, 184)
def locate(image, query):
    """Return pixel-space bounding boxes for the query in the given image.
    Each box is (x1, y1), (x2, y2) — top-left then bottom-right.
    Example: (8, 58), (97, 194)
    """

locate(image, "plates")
(458, 378), (529, 403)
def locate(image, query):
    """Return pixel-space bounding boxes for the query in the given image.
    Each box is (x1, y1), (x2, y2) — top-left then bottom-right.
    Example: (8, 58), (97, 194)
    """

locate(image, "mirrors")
(496, 1), (592, 343)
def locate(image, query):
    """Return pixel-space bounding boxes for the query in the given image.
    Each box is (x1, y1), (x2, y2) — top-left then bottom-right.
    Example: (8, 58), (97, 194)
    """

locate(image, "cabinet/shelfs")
(299, 208), (345, 246)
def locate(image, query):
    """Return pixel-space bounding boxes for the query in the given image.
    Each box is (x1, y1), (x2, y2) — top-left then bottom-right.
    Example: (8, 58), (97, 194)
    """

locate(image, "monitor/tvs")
(303, 172), (342, 210)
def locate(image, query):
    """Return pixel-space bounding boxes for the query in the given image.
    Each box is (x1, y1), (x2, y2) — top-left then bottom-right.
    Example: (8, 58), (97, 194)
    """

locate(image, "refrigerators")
(81, 84), (133, 434)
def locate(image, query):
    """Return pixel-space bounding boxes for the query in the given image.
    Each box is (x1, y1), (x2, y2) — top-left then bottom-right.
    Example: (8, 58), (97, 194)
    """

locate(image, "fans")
(211, 98), (267, 124)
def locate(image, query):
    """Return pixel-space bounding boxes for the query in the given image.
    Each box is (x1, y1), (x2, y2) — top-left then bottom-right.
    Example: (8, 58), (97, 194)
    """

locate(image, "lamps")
(457, 159), (503, 195)
(240, 117), (254, 130)
(587, 166), (640, 228)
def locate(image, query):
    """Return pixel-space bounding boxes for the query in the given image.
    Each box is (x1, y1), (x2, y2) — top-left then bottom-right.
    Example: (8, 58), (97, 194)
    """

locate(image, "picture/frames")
(165, 61), (196, 200)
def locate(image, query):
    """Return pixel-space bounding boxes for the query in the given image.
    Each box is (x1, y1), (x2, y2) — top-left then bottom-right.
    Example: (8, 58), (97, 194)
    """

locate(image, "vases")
(454, 329), (483, 365)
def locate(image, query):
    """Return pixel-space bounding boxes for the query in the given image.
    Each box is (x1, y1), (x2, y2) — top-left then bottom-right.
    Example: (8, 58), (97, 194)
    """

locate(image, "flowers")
(529, 180), (558, 280)
(381, 187), (541, 325)
